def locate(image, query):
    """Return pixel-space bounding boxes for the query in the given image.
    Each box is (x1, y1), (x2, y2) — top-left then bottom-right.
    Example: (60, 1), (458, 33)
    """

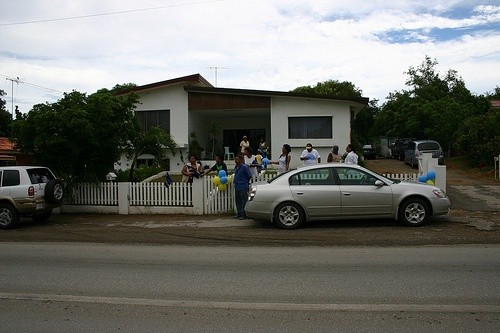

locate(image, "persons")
(201, 153), (228, 177)
(300, 143), (321, 166)
(269, 144), (291, 175)
(181, 155), (205, 205)
(233, 155), (252, 220)
(240, 136), (269, 183)
(344, 144), (359, 165)
(327, 145), (342, 162)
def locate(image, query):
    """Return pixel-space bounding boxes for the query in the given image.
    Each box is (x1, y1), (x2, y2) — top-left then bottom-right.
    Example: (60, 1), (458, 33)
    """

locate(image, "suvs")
(0, 166), (67, 230)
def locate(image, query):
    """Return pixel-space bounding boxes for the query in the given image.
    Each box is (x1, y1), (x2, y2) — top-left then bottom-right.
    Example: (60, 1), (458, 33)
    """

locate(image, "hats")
(305, 143), (312, 149)
(243, 136), (247, 138)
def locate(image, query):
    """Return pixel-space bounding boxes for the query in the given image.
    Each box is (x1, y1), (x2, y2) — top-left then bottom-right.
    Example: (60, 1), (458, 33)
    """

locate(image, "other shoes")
(233, 213), (250, 220)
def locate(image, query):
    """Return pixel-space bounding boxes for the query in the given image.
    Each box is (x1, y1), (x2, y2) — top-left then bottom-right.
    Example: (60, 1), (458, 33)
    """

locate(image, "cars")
(361, 144), (376, 160)
(243, 162), (452, 230)
(387, 137), (445, 169)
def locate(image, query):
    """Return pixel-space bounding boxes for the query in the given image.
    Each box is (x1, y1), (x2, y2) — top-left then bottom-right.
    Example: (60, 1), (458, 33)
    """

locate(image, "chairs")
(224, 147), (234, 160)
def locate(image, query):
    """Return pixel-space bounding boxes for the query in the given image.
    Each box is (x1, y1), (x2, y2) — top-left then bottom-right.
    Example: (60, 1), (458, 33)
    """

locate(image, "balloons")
(262, 158), (269, 166)
(212, 170), (231, 191)
(418, 170), (436, 185)
